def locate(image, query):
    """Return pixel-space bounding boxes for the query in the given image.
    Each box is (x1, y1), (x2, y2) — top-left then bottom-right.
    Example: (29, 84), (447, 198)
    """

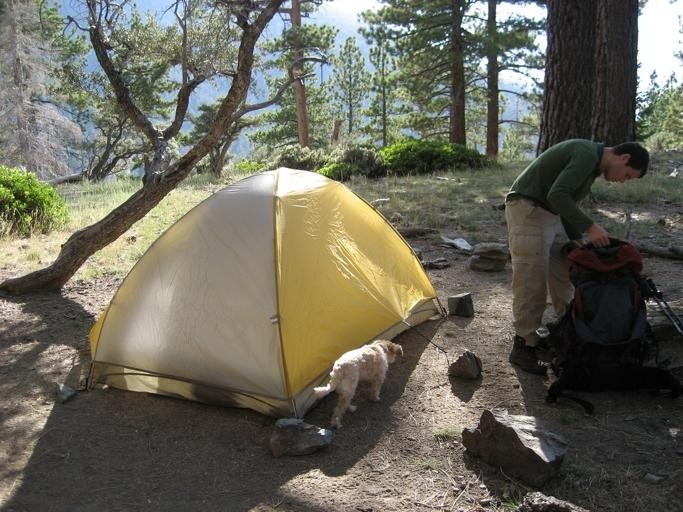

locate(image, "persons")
(503, 137), (650, 377)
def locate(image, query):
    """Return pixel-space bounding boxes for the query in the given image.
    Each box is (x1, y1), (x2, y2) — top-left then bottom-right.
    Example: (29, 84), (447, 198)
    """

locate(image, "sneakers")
(509, 334), (548, 374)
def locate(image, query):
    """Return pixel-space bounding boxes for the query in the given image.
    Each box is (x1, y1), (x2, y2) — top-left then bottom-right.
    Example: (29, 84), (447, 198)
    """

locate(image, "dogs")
(312, 339), (404, 430)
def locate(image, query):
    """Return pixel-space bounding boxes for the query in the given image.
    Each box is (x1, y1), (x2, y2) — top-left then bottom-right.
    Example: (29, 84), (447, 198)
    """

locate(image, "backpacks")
(555, 237), (661, 399)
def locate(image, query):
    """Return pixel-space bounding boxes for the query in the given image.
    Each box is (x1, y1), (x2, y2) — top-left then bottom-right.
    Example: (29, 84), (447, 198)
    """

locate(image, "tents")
(84, 166), (444, 420)
